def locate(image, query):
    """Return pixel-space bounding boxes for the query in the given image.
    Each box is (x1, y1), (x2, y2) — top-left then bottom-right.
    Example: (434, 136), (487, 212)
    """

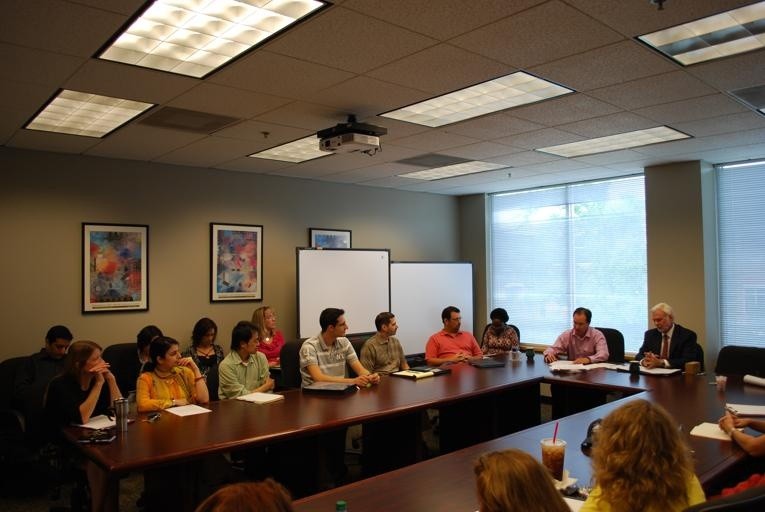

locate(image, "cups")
(628, 360), (641, 377)
(509, 351), (522, 361)
(107, 397), (130, 431)
(715, 375), (728, 392)
(525, 347), (536, 360)
(539, 437), (567, 482)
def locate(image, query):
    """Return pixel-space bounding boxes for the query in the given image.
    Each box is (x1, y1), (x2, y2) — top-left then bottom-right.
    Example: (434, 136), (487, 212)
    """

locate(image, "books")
(391, 369), (434, 380)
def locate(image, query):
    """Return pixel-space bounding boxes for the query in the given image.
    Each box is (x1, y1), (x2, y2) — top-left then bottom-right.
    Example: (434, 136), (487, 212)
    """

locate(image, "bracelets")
(728, 428), (735, 438)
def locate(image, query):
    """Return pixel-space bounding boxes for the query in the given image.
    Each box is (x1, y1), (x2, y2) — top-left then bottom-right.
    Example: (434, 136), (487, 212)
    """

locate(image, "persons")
(299, 309), (381, 494)
(475, 449), (570, 512)
(544, 308), (609, 416)
(481, 309), (519, 426)
(359, 313), (435, 469)
(425, 307), (484, 451)
(579, 400), (707, 512)
(718, 408), (765, 458)
(635, 302), (698, 372)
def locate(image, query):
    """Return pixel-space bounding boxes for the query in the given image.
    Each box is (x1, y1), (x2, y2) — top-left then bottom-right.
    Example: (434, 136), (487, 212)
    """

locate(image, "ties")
(661, 334), (670, 361)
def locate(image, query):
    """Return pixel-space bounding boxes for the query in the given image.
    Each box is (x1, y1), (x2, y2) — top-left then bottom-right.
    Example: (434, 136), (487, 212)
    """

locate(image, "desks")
(62, 349), (764, 511)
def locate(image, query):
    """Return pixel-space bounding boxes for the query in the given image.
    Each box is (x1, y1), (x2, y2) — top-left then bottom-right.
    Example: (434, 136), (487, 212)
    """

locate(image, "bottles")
(333, 500), (348, 512)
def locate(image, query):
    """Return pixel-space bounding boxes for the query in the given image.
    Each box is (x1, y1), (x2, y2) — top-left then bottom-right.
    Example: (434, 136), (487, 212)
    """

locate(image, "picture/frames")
(81, 221), (150, 315)
(308, 227), (353, 248)
(209, 221), (264, 304)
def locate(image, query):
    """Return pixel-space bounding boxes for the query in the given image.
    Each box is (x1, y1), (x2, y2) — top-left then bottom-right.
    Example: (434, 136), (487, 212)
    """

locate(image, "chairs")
(680, 485), (764, 511)
(481, 325), (522, 352)
(347, 335), (371, 382)
(715, 346), (764, 378)
(101, 343), (141, 399)
(595, 328), (626, 363)
(279, 339), (307, 389)
(207, 364), (223, 403)
(1, 357), (34, 446)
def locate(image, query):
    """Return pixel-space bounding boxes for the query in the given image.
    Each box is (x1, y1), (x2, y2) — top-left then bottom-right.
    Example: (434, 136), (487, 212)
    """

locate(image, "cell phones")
(77, 435), (94, 442)
(96, 433), (116, 443)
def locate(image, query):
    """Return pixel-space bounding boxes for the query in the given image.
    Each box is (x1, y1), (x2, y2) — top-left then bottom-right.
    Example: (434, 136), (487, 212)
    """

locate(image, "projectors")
(319, 133), (379, 154)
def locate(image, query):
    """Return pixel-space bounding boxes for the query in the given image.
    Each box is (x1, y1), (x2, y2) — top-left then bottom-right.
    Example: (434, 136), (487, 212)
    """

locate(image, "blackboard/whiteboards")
(389, 262), (477, 358)
(296, 246), (390, 338)
